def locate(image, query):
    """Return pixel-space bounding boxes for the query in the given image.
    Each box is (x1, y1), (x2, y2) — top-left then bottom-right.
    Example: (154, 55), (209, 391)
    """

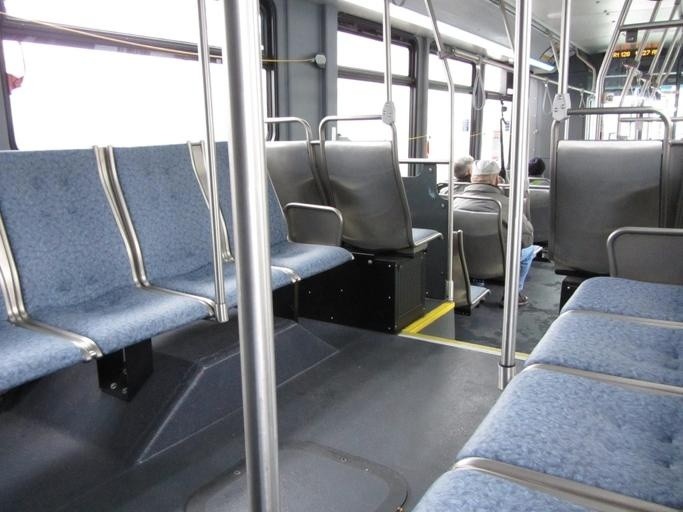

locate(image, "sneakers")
(500, 293), (529, 307)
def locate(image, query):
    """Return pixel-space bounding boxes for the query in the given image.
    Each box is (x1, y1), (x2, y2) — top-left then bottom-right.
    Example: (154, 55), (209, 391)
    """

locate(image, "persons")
(528, 157), (551, 186)
(438, 155), (534, 306)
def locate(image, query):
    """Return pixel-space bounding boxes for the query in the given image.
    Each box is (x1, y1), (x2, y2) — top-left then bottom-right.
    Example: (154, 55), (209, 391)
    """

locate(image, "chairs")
(0, 142), (357, 388)
(264, 134), (548, 310)
(415, 106), (680, 506)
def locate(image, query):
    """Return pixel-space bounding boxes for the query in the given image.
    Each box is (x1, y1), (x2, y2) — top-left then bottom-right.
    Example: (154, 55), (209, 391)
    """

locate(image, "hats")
(454, 155), (500, 180)
